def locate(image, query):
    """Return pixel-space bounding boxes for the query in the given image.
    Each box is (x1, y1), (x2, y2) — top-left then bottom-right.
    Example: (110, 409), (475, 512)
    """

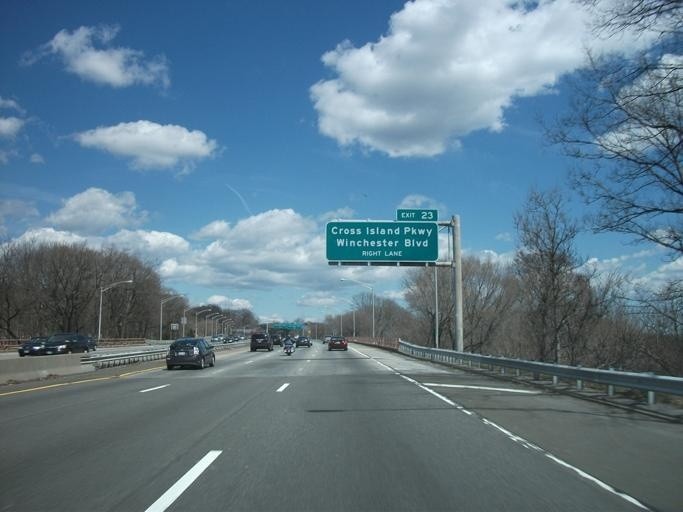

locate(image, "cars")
(323, 335), (332, 344)
(295, 336), (311, 348)
(166, 337), (216, 369)
(18, 332), (98, 356)
(327, 335), (348, 351)
(211, 334), (239, 343)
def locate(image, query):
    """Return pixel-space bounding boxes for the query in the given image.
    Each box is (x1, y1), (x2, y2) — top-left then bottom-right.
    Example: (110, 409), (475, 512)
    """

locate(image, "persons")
(284, 336), (294, 348)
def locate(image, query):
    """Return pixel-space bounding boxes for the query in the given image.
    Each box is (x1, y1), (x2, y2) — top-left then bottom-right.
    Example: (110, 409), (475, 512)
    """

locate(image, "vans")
(270, 333), (283, 347)
(250, 332), (274, 352)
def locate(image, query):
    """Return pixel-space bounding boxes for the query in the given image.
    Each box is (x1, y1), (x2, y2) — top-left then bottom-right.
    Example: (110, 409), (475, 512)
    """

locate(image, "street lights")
(160, 294), (184, 340)
(195, 309), (234, 338)
(340, 278), (375, 338)
(98, 280), (134, 339)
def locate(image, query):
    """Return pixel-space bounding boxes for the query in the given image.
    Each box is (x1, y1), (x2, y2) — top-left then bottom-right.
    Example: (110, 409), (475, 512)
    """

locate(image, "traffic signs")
(325, 219), (439, 262)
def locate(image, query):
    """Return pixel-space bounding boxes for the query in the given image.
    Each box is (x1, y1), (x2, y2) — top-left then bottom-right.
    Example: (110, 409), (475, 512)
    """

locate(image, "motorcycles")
(282, 340), (296, 356)
